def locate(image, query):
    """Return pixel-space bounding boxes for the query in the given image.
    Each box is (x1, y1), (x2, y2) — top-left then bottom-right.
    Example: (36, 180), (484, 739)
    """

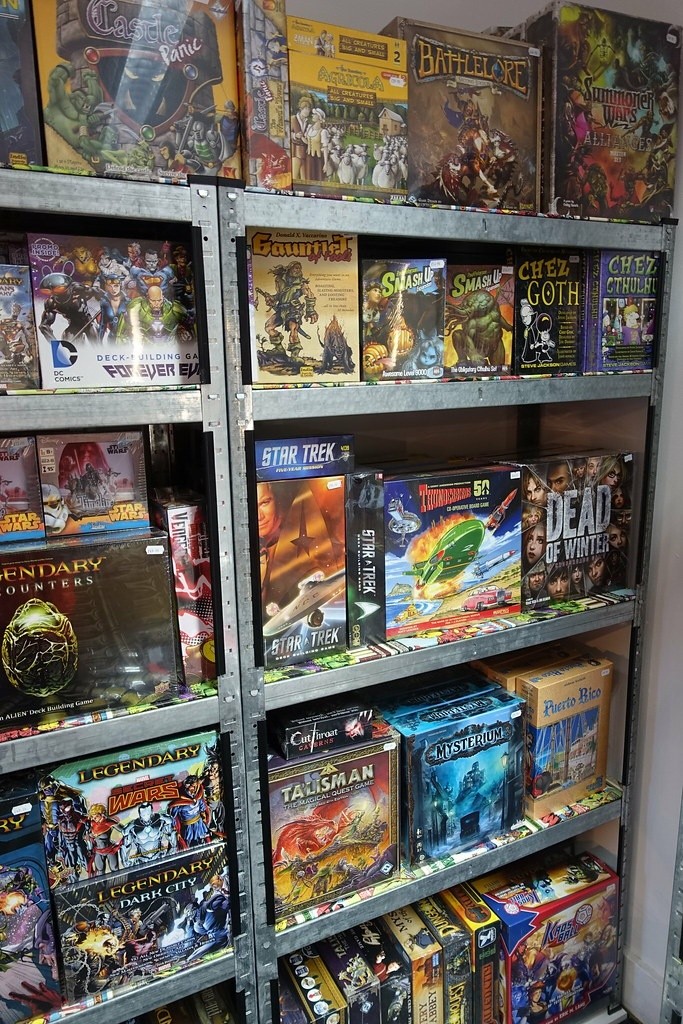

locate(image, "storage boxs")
(2, 1), (683, 1022)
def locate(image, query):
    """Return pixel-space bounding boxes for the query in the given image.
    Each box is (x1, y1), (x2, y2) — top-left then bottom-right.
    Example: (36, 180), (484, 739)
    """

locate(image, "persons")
(257, 482), (294, 605)
(521, 456), (632, 606)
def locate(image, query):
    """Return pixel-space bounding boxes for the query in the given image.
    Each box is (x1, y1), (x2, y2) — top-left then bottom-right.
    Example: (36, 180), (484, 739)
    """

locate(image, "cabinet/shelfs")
(0, 173), (260, 1024)
(213, 182), (668, 1023)
